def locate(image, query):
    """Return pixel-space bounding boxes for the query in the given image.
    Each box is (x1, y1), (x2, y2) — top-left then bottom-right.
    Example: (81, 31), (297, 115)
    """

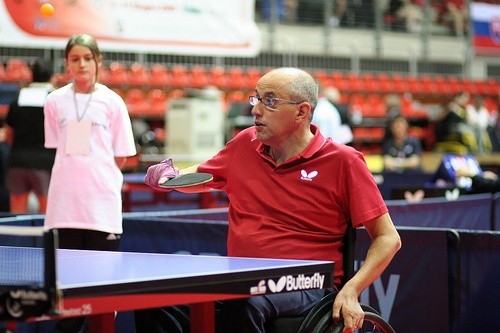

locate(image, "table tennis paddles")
(143, 171), (214, 189)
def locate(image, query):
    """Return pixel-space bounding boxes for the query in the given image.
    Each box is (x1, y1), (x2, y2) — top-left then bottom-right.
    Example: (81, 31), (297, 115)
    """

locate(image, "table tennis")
(40, 3), (54, 18)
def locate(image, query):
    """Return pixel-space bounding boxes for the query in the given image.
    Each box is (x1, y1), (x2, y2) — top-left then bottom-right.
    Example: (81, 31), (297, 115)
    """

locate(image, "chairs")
(0, 59), (500, 213)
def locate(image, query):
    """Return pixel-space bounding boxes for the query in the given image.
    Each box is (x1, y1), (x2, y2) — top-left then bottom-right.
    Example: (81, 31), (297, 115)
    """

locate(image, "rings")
(355, 325), (358, 329)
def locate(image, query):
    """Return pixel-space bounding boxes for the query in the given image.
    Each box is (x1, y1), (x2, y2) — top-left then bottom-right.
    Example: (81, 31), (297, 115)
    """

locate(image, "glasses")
(249, 96), (304, 108)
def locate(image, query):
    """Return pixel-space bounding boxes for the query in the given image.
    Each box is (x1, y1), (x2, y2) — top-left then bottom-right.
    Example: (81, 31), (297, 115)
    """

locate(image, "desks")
(116, 170), (224, 211)
(0, 228), (333, 333)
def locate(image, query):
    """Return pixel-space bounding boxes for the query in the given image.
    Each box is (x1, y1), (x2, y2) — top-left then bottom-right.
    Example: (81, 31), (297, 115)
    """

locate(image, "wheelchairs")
(202, 212), (404, 333)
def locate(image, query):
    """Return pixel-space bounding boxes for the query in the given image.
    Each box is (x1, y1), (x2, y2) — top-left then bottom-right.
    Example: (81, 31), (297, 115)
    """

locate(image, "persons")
(7, 58), (57, 214)
(143, 67), (401, 333)
(312, 86), (500, 200)
(44, 35), (137, 251)
